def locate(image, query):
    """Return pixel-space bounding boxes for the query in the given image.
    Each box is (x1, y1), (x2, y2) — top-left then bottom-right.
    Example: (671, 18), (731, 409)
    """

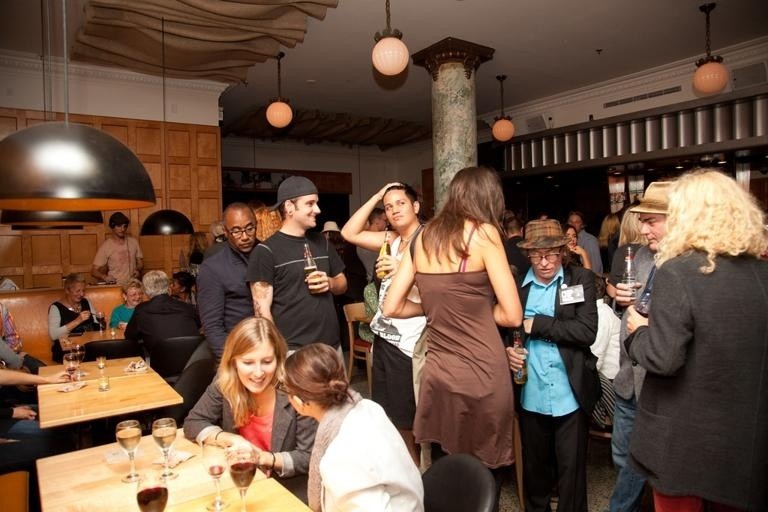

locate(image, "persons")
(0, 166), (768, 512)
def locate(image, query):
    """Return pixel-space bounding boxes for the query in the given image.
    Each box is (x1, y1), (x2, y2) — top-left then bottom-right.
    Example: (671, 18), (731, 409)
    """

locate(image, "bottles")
(373, 232), (394, 279)
(618, 247), (638, 309)
(506, 331), (528, 387)
(300, 241), (320, 297)
(623, 288), (655, 338)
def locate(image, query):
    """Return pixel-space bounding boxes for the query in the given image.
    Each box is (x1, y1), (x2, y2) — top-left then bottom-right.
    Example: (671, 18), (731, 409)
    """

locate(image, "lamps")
(371, 0), (410, 76)
(528, 113), (553, 132)
(266, 52), (292, 129)
(492, 75), (514, 142)
(693, 2), (729, 94)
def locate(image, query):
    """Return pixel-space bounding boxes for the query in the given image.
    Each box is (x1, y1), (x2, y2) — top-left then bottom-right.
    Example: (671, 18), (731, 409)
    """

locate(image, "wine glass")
(201, 439), (232, 512)
(70, 341), (88, 377)
(115, 419), (147, 484)
(95, 311), (105, 333)
(135, 471), (170, 511)
(149, 417), (181, 484)
(227, 443), (259, 512)
(63, 353), (79, 388)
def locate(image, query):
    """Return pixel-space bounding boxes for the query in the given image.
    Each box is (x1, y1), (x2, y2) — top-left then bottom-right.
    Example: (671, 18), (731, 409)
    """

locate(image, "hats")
(267, 175), (320, 212)
(516, 220), (571, 249)
(629, 180), (675, 215)
(321, 221), (340, 232)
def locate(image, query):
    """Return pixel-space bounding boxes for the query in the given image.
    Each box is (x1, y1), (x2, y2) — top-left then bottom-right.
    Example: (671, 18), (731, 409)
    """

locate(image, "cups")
(97, 375), (110, 392)
(95, 353), (107, 369)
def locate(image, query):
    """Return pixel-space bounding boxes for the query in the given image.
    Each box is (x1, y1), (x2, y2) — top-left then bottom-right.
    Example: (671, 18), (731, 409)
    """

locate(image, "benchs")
(0, 285), (149, 512)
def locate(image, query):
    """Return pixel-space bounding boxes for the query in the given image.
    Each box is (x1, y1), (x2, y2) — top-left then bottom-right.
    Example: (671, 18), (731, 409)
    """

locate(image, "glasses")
(224, 225), (257, 239)
(276, 383), (310, 408)
(526, 247), (563, 265)
